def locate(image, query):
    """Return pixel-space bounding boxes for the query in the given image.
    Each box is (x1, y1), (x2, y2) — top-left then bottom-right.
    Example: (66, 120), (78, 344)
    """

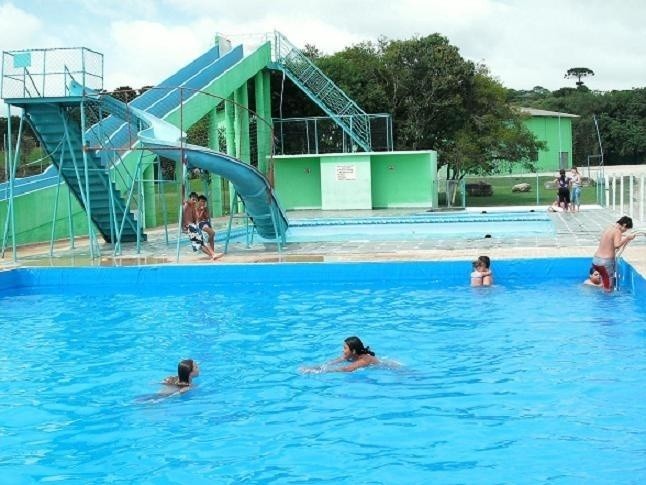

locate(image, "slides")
(68, 80), (289, 239)
(0, 32), (272, 250)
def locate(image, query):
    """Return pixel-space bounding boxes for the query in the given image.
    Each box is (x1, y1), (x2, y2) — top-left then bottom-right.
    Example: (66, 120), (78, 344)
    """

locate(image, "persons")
(468, 260), (492, 287)
(179, 191), (223, 259)
(592, 214), (636, 292)
(581, 268), (603, 288)
(194, 195), (216, 251)
(567, 165), (583, 213)
(298, 334), (402, 373)
(477, 256), (492, 286)
(131, 359), (200, 403)
(552, 168), (572, 210)
